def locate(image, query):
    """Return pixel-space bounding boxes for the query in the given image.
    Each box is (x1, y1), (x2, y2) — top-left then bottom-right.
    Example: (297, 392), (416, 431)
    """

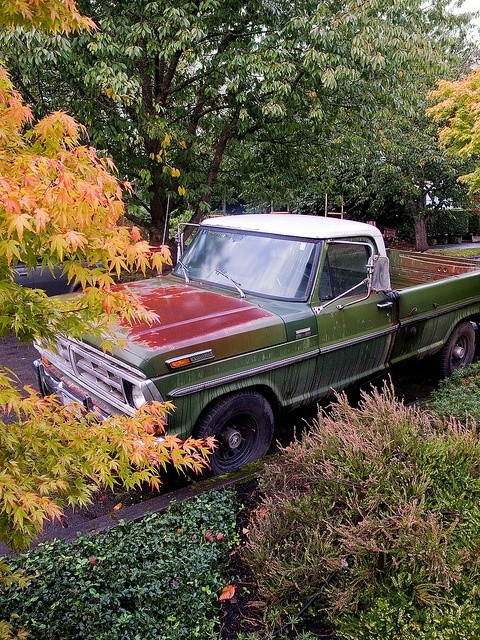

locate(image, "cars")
(8, 252), (107, 297)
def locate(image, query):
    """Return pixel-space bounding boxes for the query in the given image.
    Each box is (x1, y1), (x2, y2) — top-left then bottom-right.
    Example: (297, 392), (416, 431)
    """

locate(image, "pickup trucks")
(31, 213), (480, 475)
